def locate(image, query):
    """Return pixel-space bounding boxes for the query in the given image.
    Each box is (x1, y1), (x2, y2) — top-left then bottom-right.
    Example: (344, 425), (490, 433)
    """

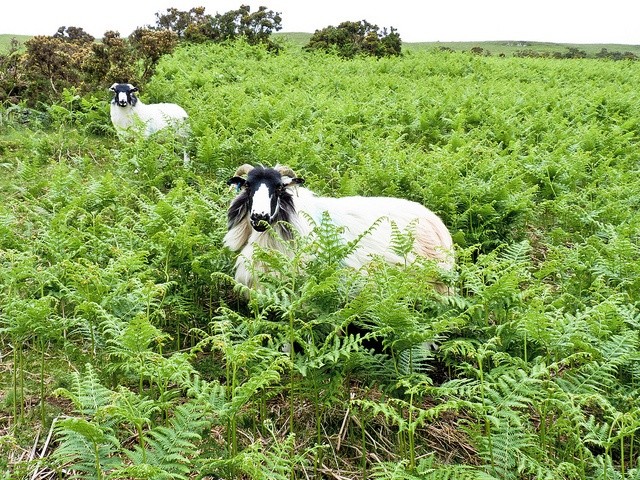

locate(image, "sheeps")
(108, 83), (189, 144)
(221, 162), (456, 308)
